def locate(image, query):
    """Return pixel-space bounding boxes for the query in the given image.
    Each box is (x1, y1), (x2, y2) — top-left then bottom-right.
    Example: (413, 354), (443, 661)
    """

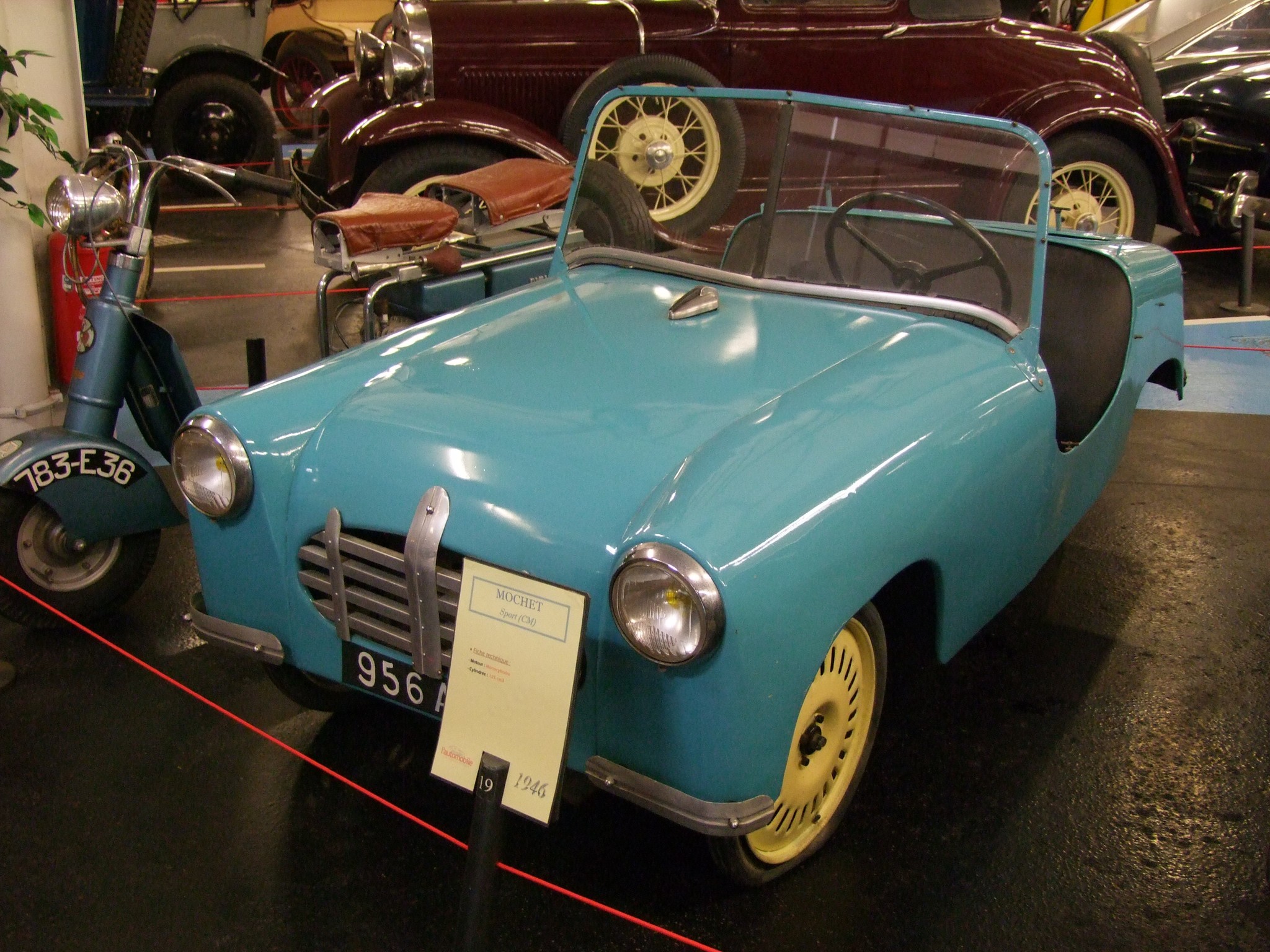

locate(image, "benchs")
(725, 213), (1133, 442)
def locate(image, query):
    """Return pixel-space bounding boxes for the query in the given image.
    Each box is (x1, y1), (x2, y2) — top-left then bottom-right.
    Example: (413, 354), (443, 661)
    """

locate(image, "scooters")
(2, 131), (582, 631)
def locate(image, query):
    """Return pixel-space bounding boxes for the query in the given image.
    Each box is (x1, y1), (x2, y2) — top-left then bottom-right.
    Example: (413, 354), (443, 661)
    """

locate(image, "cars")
(1152, 0), (1270, 237)
(291, 0), (1200, 245)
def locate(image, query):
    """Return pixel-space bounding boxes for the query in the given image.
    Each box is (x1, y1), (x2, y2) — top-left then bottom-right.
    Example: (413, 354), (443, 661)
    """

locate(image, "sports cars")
(169, 81), (1190, 888)
